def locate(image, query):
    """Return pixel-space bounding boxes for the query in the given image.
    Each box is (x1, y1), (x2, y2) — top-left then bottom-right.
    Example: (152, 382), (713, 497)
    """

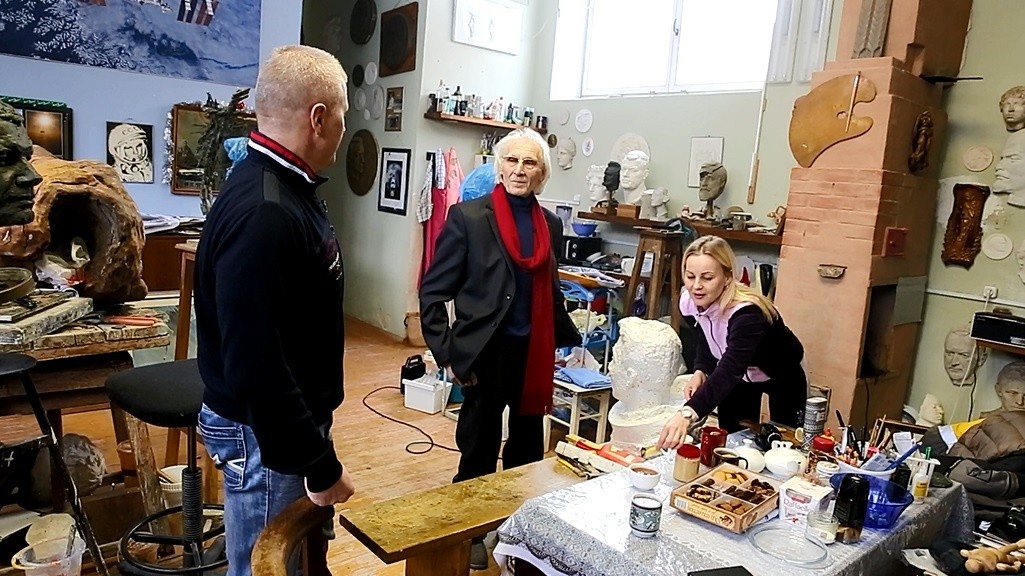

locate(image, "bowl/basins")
(571, 216), (598, 237)
(829, 471), (915, 529)
(835, 458), (897, 481)
(629, 464), (661, 490)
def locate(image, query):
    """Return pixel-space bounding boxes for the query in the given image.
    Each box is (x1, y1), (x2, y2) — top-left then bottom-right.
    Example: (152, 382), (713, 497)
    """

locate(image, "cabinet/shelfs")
(141, 234), (202, 291)
(553, 277), (617, 421)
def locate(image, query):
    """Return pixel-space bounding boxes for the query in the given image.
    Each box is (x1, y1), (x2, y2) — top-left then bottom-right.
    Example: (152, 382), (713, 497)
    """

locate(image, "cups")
(701, 426), (729, 468)
(11, 535), (86, 576)
(621, 258), (633, 276)
(159, 464), (189, 509)
(673, 444), (701, 483)
(803, 509), (839, 545)
(555, 205), (572, 236)
(711, 448), (748, 470)
(629, 493), (663, 539)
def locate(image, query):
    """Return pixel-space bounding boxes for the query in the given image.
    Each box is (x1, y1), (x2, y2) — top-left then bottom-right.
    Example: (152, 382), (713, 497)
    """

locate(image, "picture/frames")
(378, 147), (411, 216)
(169, 104), (257, 196)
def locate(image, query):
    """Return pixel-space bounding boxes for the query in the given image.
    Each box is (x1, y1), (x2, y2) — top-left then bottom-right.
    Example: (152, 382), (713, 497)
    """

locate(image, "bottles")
(807, 437), (835, 473)
(428, 85), (547, 129)
(831, 473), (870, 545)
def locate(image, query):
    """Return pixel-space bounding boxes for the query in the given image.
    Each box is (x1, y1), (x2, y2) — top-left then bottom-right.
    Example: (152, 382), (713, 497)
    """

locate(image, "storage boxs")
(402, 374), (454, 415)
(670, 461), (783, 534)
(779, 475), (834, 526)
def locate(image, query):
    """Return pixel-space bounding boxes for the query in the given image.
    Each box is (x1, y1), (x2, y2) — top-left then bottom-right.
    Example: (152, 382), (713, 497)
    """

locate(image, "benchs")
(339, 453), (591, 576)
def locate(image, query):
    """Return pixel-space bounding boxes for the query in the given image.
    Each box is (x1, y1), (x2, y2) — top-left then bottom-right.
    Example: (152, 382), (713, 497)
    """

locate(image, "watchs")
(677, 408), (696, 423)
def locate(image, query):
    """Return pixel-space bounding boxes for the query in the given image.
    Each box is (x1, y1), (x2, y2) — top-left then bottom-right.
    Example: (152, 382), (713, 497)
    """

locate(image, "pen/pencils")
(925, 446), (932, 460)
(835, 409), (924, 471)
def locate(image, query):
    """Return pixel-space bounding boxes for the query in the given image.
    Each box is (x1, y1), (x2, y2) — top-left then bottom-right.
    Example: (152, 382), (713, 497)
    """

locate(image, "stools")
(543, 378), (612, 455)
(0, 350), (110, 576)
(103, 356), (229, 576)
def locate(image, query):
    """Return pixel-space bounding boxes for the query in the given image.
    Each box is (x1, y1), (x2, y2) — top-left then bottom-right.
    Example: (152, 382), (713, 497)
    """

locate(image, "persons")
(557, 138), (576, 170)
(978, 359), (1025, 419)
(585, 165), (608, 212)
(602, 161), (621, 192)
(656, 234), (808, 451)
(992, 130), (1025, 209)
(944, 324), (988, 385)
(620, 149), (650, 205)
(194, 44), (356, 575)
(999, 86), (1024, 133)
(650, 186), (670, 206)
(607, 316), (682, 450)
(915, 394), (943, 427)
(0, 98), (42, 228)
(419, 128), (583, 483)
(698, 160), (726, 201)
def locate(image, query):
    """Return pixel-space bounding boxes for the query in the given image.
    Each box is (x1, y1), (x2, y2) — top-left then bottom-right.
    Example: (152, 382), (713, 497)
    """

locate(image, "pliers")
(83, 315), (158, 326)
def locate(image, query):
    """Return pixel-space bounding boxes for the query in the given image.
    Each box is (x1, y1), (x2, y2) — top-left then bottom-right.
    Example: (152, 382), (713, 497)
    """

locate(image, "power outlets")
(984, 286), (998, 298)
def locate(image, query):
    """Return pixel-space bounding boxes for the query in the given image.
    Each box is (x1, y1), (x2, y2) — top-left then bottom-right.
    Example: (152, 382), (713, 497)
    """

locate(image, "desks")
(624, 227), (683, 334)
(492, 421), (977, 576)
(0, 305), (173, 576)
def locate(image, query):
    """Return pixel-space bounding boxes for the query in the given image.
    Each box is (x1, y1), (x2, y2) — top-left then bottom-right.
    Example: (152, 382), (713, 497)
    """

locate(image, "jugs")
(733, 438), (765, 473)
(764, 440), (807, 476)
